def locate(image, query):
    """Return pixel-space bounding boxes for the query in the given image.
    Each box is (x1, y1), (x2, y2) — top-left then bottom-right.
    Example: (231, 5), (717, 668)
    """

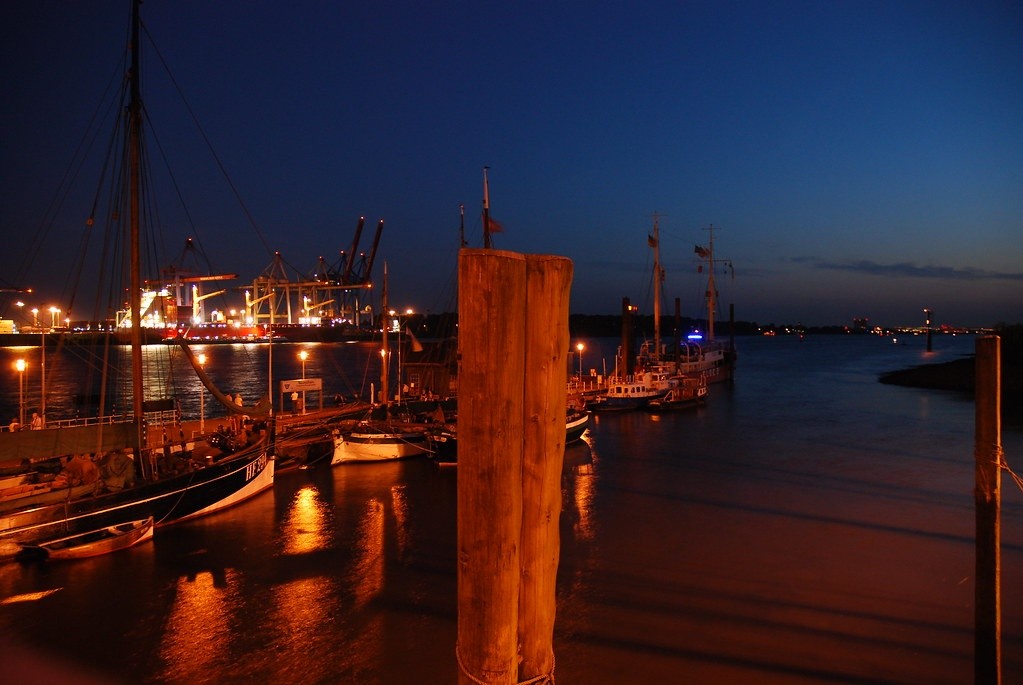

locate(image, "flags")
(648, 236), (654, 247)
(483, 217), (501, 232)
(406, 328), (422, 352)
(695, 245), (709, 257)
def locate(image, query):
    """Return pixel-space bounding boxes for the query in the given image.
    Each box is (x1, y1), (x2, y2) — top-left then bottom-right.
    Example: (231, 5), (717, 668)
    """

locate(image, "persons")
(421, 390), (433, 400)
(335, 393), (347, 403)
(31, 413), (41, 430)
(10, 419), (19, 432)
(362, 318), (368, 326)
(217, 426), (235, 437)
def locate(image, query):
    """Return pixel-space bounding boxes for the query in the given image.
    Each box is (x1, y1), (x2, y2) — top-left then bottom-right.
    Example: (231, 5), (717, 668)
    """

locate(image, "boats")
(36, 514), (155, 560)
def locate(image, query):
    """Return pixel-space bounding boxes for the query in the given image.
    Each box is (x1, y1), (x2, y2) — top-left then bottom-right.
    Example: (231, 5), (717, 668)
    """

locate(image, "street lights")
(32, 307), (38, 326)
(196, 353), (206, 435)
(50, 306), (57, 329)
(575, 342), (585, 384)
(16, 358), (26, 429)
(297, 349), (310, 413)
(56, 309), (61, 326)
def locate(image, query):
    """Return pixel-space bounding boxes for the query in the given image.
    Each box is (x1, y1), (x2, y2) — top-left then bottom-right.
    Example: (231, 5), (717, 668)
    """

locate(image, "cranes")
(229, 213), (384, 329)
(142, 236), (238, 328)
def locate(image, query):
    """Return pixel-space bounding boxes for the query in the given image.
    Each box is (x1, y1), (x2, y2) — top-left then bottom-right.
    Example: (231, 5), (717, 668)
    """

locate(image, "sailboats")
(583, 210), (739, 413)
(0, 0), (280, 558)
(330, 259), (445, 466)
(427, 167), (589, 457)
